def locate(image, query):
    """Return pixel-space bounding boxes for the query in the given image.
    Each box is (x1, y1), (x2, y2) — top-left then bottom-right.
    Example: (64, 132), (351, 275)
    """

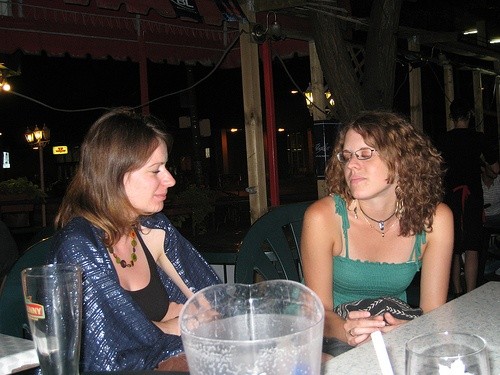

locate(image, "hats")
(450, 98), (474, 114)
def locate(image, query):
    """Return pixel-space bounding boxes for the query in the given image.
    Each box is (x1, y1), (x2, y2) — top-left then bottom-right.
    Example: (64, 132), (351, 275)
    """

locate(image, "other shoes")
(453, 291), (467, 297)
(484, 263), (500, 276)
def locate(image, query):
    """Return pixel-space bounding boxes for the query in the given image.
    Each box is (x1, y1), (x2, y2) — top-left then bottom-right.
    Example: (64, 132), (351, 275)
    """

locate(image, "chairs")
(235, 200), (316, 285)
(0, 232), (54, 337)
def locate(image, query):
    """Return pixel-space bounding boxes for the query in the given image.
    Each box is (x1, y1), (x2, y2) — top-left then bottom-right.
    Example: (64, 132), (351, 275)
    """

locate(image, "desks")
(320, 280), (500, 375)
(477, 213), (500, 287)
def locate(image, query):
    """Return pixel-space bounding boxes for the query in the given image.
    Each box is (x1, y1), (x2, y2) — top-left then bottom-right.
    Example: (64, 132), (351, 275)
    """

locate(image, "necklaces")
(353, 199), (400, 238)
(109, 229), (138, 268)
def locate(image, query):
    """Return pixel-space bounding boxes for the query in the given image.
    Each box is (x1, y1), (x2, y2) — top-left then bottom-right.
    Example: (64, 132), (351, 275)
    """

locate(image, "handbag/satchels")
(336, 297), (423, 320)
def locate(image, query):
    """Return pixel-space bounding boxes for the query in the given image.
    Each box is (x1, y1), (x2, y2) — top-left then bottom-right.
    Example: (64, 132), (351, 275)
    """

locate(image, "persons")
(39, 108), (229, 374)
(430, 98), (500, 293)
(280, 111), (454, 375)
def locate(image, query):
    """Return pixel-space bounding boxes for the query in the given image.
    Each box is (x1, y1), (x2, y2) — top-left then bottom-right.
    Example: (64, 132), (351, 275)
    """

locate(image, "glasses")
(336, 148), (381, 162)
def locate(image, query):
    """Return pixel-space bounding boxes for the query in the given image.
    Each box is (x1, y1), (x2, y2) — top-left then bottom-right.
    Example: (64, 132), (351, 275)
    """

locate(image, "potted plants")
(0, 175), (49, 232)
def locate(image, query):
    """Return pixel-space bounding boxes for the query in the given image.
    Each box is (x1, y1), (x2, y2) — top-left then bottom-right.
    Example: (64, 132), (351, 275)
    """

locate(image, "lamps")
(250, 10), (283, 42)
(423, 42), (451, 66)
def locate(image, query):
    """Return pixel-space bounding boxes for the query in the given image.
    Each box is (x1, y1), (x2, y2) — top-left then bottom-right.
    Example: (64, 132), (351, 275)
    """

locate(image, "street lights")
(24, 122), (51, 228)
(305, 81), (335, 177)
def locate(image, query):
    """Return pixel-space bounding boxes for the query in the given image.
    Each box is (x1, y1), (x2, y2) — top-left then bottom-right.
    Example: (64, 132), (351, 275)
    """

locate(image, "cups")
(404, 330), (490, 375)
(21, 263), (82, 375)
(231, 230), (243, 253)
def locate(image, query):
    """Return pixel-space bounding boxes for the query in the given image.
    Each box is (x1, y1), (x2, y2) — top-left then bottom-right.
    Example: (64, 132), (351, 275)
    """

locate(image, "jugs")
(179, 280), (326, 374)
